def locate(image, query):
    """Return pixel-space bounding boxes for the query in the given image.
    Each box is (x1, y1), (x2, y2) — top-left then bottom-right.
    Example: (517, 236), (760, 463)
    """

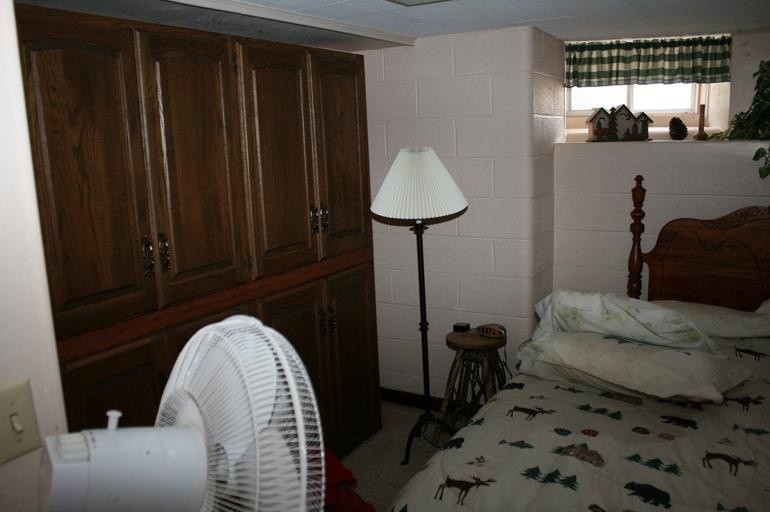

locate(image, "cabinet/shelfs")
(231, 34), (372, 283)
(62, 300), (249, 435)
(13, 0), (248, 343)
(258, 261), (380, 461)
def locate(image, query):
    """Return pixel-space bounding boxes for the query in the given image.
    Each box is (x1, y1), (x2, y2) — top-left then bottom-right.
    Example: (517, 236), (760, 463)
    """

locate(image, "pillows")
(511, 288), (755, 406)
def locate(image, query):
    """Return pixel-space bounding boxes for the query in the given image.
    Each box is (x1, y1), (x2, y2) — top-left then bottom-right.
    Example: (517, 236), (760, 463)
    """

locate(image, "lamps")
(368, 146), (469, 466)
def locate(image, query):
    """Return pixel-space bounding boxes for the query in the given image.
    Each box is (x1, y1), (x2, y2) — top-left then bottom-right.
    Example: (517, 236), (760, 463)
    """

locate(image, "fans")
(36, 314), (328, 512)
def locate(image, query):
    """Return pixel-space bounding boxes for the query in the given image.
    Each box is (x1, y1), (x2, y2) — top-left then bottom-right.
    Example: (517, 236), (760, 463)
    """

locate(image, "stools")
(430, 327), (507, 443)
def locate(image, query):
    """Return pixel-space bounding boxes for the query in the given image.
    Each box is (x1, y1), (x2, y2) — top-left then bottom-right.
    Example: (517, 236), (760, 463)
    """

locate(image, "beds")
(387, 174), (767, 509)
